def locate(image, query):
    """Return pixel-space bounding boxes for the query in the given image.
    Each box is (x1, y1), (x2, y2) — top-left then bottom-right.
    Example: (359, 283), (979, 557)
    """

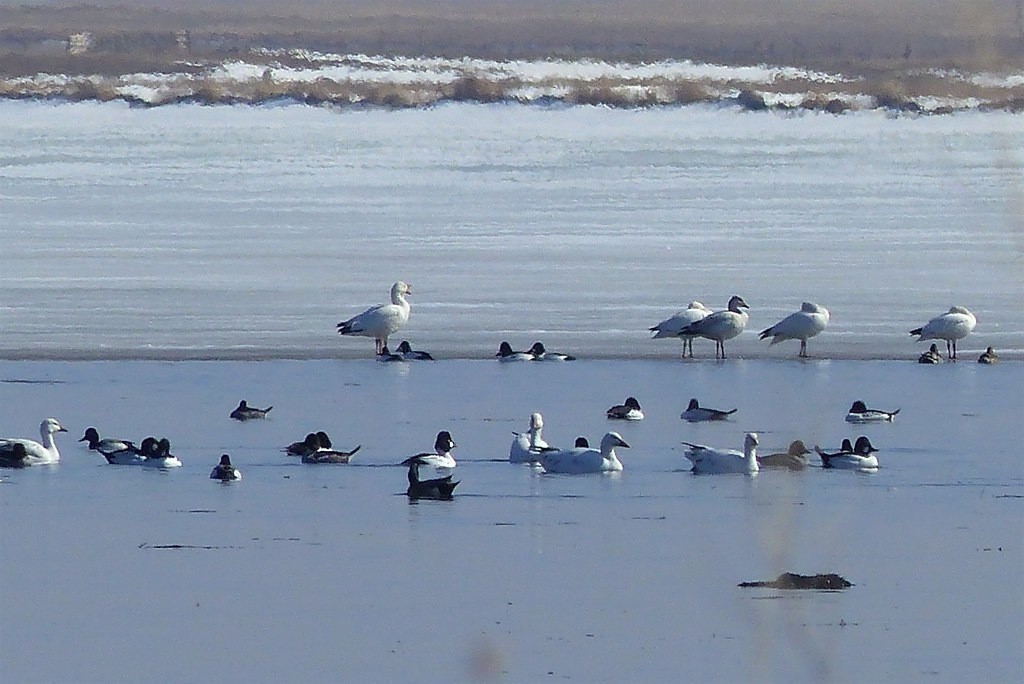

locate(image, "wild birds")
(757, 301), (830, 357)
(909, 306), (976, 360)
(918, 343), (946, 366)
(977, 346), (999, 365)
(230, 400), (273, 423)
(815, 436), (880, 470)
(279, 430), (361, 465)
(379, 341), (434, 362)
(510, 413), (630, 476)
(845, 400), (902, 423)
(495, 341), (543, 362)
(676, 293), (750, 359)
(528, 341), (577, 363)
(647, 301), (715, 359)
(607, 397), (645, 421)
(77, 427), (182, 468)
(0, 418), (68, 469)
(680, 398), (738, 423)
(407, 461), (462, 504)
(399, 431), (458, 470)
(756, 439), (812, 471)
(681, 432), (759, 478)
(334, 280), (411, 361)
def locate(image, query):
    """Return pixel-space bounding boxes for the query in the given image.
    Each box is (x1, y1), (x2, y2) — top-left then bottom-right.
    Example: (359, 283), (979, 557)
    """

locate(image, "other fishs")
(210, 454), (242, 484)
